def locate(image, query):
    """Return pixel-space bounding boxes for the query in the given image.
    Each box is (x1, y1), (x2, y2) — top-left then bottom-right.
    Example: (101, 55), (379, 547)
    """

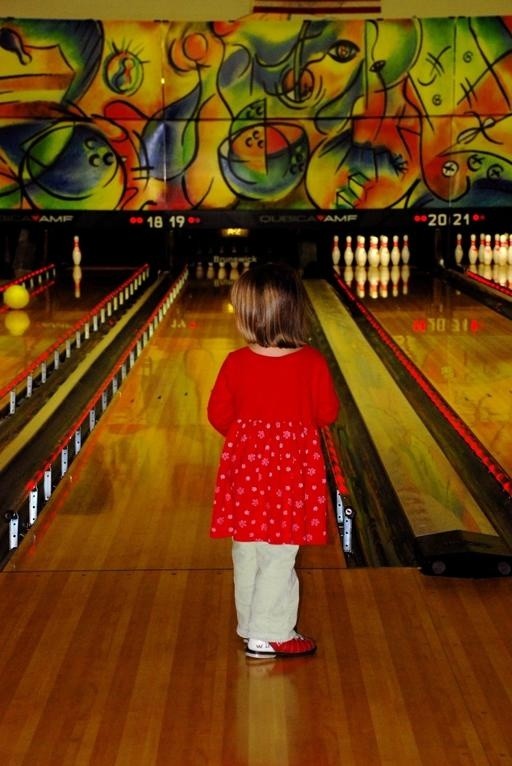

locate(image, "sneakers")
(244, 633), (316, 659)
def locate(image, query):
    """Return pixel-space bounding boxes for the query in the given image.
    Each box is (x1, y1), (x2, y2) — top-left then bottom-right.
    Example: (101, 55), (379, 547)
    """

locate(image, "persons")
(200, 257), (341, 662)
